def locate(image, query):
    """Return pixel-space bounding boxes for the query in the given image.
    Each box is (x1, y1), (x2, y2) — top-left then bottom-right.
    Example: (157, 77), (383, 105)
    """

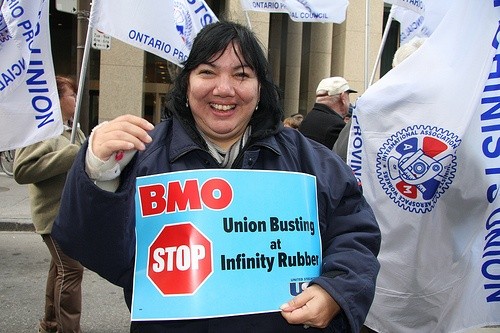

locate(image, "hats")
(316, 76), (358, 97)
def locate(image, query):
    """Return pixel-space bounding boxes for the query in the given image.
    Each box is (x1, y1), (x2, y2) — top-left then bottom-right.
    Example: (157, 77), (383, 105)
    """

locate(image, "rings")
(303, 324), (309, 328)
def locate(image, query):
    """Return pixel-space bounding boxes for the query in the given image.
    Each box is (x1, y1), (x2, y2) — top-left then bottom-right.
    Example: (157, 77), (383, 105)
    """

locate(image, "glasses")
(59, 92), (76, 102)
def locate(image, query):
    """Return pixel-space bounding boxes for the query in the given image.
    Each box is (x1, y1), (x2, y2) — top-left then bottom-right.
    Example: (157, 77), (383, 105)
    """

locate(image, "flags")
(89, 0), (220, 69)
(346, 0), (500, 333)
(0, 0), (64, 151)
(241, 0), (349, 24)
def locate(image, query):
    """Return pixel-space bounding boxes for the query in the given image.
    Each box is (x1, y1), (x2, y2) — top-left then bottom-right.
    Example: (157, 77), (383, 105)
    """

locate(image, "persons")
(331, 37), (424, 163)
(284, 113), (303, 130)
(51, 19), (381, 333)
(298, 76), (358, 150)
(13, 75), (87, 333)
(343, 103), (356, 123)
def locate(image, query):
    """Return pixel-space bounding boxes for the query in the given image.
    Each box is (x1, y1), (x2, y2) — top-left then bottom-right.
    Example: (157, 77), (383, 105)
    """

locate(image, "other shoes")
(38, 317), (58, 333)
